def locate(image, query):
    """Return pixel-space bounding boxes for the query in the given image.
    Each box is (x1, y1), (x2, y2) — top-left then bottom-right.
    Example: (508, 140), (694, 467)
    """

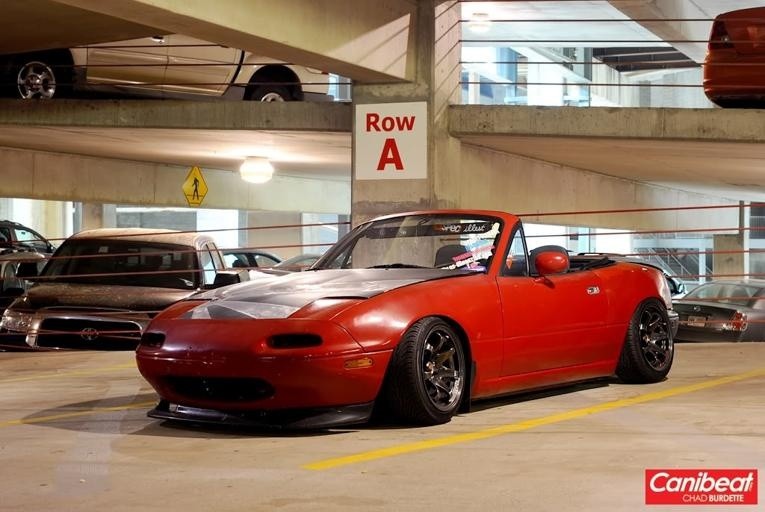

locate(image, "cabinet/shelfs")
(433, 245), (468, 270)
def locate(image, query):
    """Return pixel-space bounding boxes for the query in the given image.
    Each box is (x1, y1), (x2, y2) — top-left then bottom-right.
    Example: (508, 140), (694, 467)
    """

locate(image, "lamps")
(239, 154), (275, 184)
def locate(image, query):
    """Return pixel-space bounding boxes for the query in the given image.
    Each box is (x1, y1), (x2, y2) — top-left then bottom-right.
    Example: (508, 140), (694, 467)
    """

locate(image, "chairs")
(528, 244), (571, 275)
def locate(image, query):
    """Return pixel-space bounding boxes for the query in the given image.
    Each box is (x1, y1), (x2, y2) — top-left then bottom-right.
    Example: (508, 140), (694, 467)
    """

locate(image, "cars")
(578, 251), (688, 307)
(0, 219), (343, 309)
(700, 5), (765, 109)
(5, 33), (332, 102)
(670, 277), (765, 344)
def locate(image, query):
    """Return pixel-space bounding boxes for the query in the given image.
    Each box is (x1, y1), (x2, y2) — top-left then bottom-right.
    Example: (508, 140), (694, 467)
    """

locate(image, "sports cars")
(133, 205), (687, 434)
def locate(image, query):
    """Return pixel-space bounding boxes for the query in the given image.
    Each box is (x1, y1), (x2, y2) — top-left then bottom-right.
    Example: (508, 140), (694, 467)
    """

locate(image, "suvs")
(0, 225), (232, 354)
